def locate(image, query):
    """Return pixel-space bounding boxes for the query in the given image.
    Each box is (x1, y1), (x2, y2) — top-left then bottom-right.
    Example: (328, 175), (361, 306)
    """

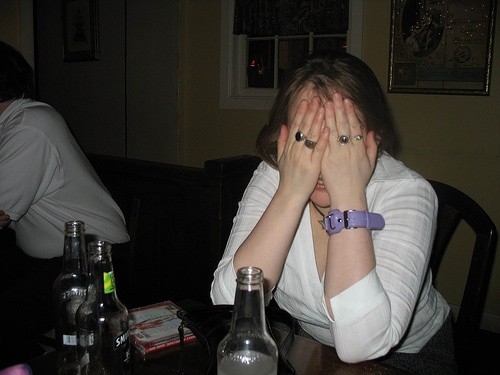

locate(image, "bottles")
(217, 266), (278, 375)
(51, 221), (101, 375)
(74, 240), (131, 375)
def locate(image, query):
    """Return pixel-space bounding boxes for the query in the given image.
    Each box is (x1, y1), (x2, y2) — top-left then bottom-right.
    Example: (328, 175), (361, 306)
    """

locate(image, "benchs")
(0, 152), (236, 357)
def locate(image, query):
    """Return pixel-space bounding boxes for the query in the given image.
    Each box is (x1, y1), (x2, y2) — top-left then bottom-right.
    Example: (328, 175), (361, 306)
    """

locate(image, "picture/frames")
(387, 0), (498, 97)
(61, 0), (102, 64)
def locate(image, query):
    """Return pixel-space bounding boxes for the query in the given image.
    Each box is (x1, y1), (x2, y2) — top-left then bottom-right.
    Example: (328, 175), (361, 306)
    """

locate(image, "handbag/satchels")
(177, 305), (296, 375)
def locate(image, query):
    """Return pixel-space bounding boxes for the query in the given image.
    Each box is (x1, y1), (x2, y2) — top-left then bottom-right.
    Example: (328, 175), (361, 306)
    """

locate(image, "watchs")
(324, 208), (385, 235)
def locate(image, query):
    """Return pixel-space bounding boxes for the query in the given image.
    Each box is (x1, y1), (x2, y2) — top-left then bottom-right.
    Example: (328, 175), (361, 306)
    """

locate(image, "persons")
(209, 51), (457, 375)
(0, 41), (132, 336)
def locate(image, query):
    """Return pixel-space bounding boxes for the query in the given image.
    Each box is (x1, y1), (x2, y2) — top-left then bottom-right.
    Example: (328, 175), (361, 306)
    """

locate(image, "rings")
(305, 140), (317, 149)
(339, 135), (350, 143)
(352, 135), (363, 140)
(296, 131), (305, 141)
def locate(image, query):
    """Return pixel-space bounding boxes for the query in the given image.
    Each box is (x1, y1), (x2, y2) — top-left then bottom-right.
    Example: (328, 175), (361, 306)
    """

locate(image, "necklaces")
(313, 201), (324, 217)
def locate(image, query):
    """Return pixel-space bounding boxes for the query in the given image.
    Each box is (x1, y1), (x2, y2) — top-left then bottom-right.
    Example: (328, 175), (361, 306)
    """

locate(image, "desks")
(24, 324), (217, 375)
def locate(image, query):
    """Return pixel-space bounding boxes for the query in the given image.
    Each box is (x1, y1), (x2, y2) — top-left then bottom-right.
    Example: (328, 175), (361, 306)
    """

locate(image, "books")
(127, 299), (196, 354)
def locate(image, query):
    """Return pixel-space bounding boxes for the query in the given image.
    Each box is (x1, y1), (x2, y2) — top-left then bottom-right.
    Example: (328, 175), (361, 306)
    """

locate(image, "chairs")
(228, 163), (497, 375)
